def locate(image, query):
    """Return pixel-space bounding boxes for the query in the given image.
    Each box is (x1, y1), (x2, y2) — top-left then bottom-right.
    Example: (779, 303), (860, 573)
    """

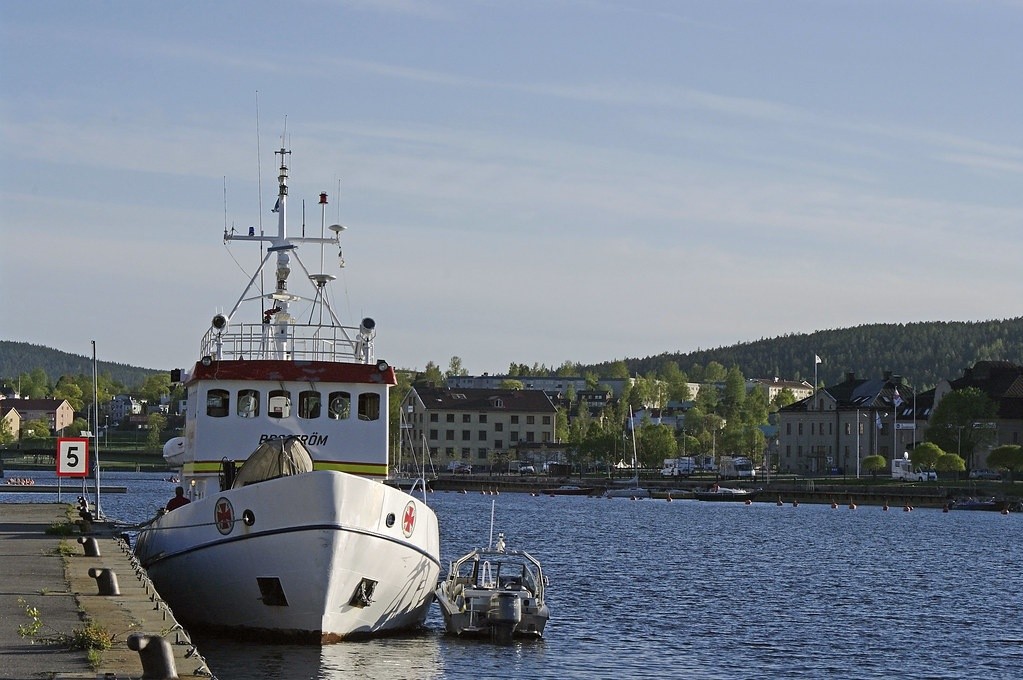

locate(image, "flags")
(816, 355), (822, 363)
(877, 413), (883, 429)
(894, 390), (903, 406)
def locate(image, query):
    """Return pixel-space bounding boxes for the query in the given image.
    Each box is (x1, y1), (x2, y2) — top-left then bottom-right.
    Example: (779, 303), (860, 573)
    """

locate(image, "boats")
(441, 499), (551, 638)
(134, 122), (438, 636)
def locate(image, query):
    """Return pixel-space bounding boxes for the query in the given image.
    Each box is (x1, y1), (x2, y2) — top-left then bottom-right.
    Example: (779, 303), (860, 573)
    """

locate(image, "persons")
(713, 483), (720, 493)
(167, 486), (190, 511)
(6, 475), (33, 485)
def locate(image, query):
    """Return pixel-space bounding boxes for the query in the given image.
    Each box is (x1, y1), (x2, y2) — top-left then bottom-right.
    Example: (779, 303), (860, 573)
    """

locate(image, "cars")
(661, 457), (695, 479)
(893, 459), (937, 483)
(516, 462), (535, 473)
(718, 457), (754, 479)
